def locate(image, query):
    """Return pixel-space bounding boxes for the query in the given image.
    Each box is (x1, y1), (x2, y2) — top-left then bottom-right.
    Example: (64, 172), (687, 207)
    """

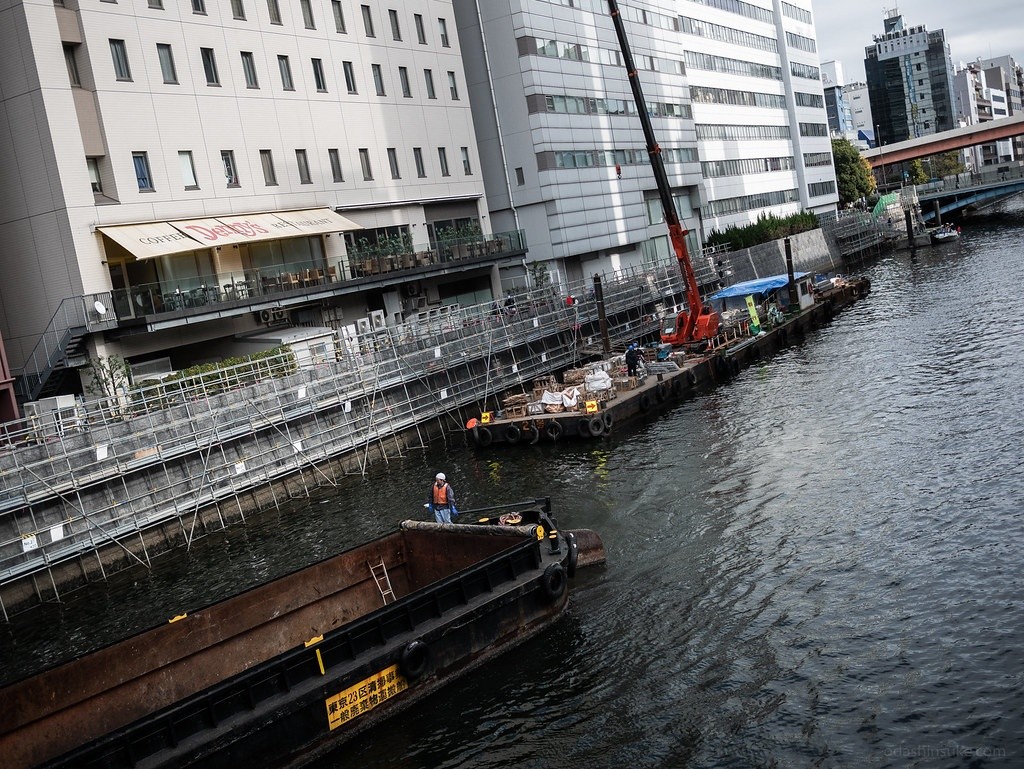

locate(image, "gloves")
(452, 509), (458, 515)
(429, 504), (432, 512)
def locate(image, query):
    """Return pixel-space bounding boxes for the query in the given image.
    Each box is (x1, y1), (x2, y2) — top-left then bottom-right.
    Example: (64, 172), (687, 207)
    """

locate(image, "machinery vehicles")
(608, 1), (725, 352)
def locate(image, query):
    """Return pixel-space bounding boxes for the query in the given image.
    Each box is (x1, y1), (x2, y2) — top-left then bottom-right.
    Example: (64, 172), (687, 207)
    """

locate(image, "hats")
(629, 345), (633, 350)
(436, 473), (446, 481)
(632, 341), (639, 347)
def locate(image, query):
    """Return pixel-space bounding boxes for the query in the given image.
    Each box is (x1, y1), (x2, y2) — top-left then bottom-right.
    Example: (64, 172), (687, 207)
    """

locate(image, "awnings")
(90, 206), (366, 261)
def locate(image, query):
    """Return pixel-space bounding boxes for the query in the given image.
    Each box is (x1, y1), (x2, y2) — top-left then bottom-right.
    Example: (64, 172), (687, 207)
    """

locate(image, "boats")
(468, 236), (873, 446)
(930, 228), (962, 245)
(1, 496), (606, 769)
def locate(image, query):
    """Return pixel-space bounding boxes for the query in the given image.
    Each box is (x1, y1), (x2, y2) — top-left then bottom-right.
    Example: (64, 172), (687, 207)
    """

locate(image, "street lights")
(877, 124), (887, 195)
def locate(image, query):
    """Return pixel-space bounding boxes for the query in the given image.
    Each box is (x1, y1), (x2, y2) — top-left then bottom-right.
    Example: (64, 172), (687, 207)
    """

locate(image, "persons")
(504, 294), (515, 307)
(588, 289), (595, 302)
(423, 472), (459, 525)
(625, 345), (639, 377)
(570, 295), (579, 318)
